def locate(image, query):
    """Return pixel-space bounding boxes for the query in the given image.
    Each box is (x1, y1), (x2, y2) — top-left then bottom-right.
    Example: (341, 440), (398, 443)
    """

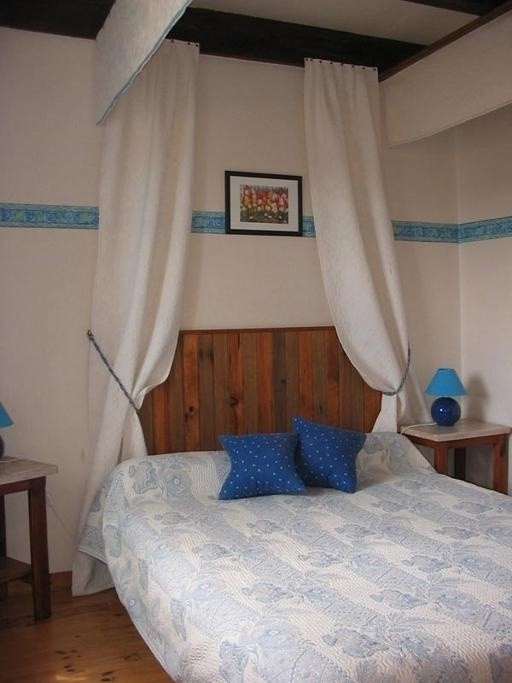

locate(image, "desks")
(0, 456), (60, 625)
(397, 418), (512, 496)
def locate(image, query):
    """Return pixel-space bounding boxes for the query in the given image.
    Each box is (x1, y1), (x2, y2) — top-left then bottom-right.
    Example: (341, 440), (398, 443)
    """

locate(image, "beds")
(80, 324), (512, 683)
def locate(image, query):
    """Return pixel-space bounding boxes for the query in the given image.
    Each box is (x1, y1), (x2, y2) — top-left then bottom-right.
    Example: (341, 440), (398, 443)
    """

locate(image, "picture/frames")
(225, 170), (304, 236)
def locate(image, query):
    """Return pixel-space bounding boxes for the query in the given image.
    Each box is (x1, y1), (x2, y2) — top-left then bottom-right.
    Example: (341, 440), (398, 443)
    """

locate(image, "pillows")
(209, 418), (368, 500)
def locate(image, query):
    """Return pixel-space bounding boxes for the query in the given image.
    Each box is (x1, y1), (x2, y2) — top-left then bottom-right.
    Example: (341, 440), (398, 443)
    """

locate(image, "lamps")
(0, 402), (15, 460)
(423, 368), (468, 427)
